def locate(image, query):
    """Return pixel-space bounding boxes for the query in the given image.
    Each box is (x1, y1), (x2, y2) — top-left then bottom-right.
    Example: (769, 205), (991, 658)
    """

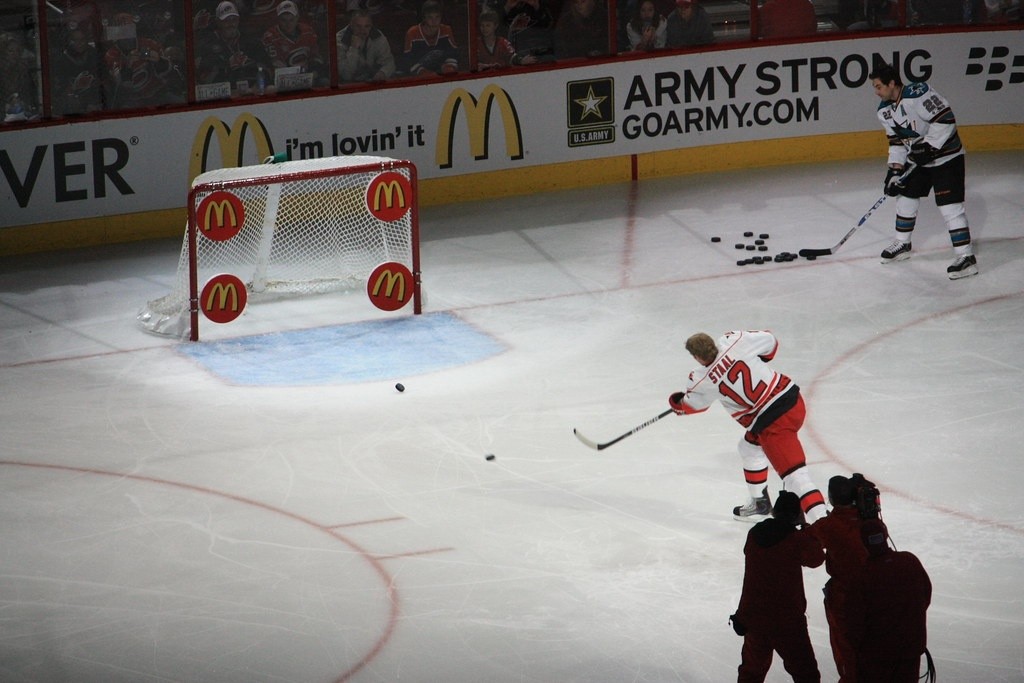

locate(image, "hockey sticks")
(798, 196), (894, 259)
(571, 407), (676, 451)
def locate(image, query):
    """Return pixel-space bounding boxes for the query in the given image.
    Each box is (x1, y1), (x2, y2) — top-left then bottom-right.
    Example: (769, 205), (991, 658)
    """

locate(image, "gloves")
(668, 392), (685, 416)
(905, 142), (932, 166)
(72, 71), (96, 95)
(884, 167), (905, 197)
(139, 47), (160, 64)
(108, 62), (121, 78)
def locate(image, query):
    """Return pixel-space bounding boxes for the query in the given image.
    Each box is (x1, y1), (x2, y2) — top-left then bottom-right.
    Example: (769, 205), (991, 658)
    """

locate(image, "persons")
(868, 62), (980, 280)
(864, 0), (1024, 28)
(730, 489), (825, 683)
(668, 329), (832, 525)
(0, 1), (720, 124)
(802, 472), (937, 683)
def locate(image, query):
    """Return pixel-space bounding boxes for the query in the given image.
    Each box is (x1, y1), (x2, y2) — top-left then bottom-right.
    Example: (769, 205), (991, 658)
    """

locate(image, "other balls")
(710, 230), (817, 265)
(485, 452), (497, 463)
(394, 382), (405, 392)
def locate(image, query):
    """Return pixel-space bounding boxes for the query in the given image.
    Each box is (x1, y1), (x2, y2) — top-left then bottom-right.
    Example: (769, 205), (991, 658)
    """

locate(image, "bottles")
(256, 67), (265, 95)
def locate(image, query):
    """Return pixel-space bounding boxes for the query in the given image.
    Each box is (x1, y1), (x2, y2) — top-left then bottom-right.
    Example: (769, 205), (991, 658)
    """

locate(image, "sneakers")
(732, 486), (774, 516)
(881, 241), (912, 258)
(947, 254), (977, 272)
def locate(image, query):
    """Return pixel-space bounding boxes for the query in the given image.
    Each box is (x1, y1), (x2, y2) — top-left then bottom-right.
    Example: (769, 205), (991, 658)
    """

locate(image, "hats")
(685, 333), (717, 359)
(676, 0), (697, 6)
(216, 2), (240, 21)
(276, 1), (298, 16)
